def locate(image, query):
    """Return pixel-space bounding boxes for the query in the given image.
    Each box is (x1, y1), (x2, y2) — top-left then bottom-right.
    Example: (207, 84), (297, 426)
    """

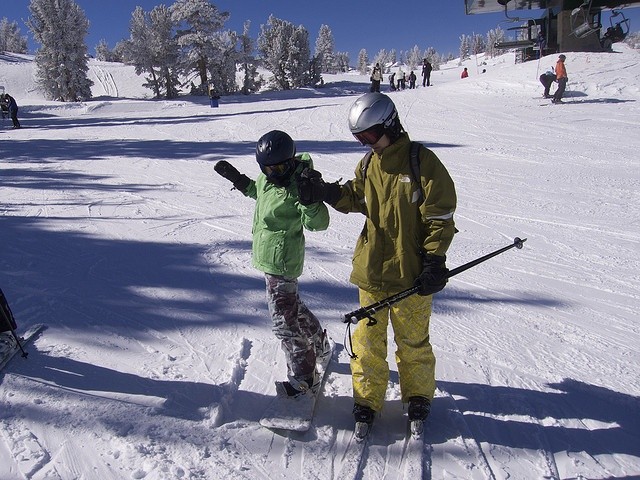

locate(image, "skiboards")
(540, 102), (570, 106)
(337, 422), (424, 480)
(0, 322), (44, 372)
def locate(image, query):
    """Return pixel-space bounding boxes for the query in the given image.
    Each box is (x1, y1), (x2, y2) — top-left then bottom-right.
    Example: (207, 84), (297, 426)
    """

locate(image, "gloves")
(214, 161), (240, 183)
(297, 168), (342, 208)
(415, 253), (448, 296)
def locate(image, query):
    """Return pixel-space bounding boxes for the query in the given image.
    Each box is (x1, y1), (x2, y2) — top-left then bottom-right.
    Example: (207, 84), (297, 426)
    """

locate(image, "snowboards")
(259, 338), (336, 432)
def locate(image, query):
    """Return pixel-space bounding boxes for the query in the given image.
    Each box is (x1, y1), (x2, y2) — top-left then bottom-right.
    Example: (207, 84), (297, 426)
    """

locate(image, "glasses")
(259, 160), (293, 177)
(353, 106), (398, 146)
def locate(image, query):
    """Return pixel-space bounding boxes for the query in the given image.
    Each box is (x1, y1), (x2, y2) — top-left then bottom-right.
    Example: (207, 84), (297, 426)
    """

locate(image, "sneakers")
(354, 404), (374, 423)
(552, 98), (563, 103)
(407, 395), (431, 420)
(280, 380), (321, 397)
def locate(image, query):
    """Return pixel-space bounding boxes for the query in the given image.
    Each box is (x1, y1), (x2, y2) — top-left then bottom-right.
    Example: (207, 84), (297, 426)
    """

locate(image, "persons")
(408, 70), (417, 89)
(552, 54), (569, 104)
(460, 67), (469, 79)
(540, 72), (558, 97)
(213, 127), (332, 400)
(319, 91), (458, 424)
(421, 58), (433, 87)
(388, 72), (396, 87)
(370, 62), (383, 93)
(4, 93), (20, 130)
(209, 86), (220, 100)
(396, 66), (404, 90)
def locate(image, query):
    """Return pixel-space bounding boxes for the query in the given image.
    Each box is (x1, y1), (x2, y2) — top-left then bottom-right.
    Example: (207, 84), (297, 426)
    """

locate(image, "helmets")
(256, 130), (296, 166)
(349, 91), (398, 145)
(559, 55), (566, 61)
